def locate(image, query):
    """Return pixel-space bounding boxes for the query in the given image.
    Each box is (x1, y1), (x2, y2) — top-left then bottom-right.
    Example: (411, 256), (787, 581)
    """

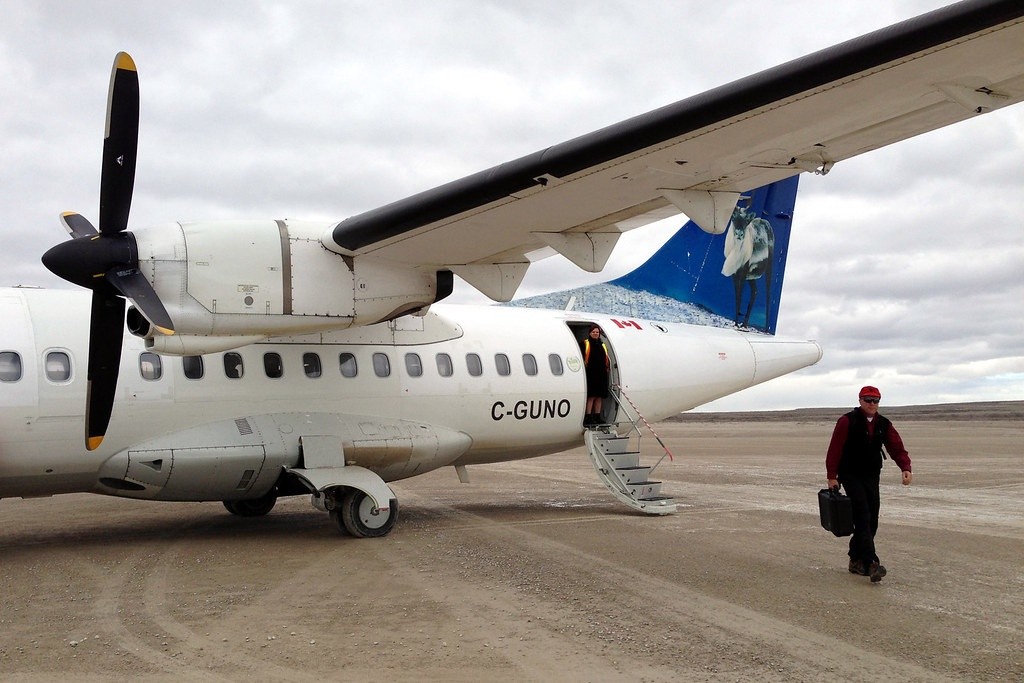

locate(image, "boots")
(583, 414), (596, 428)
(594, 413), (606, 424)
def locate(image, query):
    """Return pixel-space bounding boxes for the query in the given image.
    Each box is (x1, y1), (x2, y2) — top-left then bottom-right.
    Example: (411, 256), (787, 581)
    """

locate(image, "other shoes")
(848, 561), (870, 576)
(870, 566), (886, 582)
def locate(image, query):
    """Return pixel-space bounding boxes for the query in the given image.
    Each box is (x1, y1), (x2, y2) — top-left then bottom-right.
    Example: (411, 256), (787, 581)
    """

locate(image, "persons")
(826, 385), (912, 582)
(581, 324), (609, 425)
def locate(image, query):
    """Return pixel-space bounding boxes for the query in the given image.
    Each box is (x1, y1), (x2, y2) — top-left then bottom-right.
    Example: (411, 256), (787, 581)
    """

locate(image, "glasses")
(860, 397), (880, 404)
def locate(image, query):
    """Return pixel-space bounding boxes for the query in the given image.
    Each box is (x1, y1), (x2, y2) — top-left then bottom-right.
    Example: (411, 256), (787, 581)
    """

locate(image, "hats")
(859, 386), (881, 399)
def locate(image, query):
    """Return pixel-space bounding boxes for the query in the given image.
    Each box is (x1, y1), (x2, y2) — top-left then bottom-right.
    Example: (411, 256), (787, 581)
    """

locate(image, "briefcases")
(817, 485), (854, 537)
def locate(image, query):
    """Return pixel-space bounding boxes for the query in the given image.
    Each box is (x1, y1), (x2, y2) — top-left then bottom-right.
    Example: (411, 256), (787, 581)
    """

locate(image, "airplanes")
(0, 0), (1024, 542)
